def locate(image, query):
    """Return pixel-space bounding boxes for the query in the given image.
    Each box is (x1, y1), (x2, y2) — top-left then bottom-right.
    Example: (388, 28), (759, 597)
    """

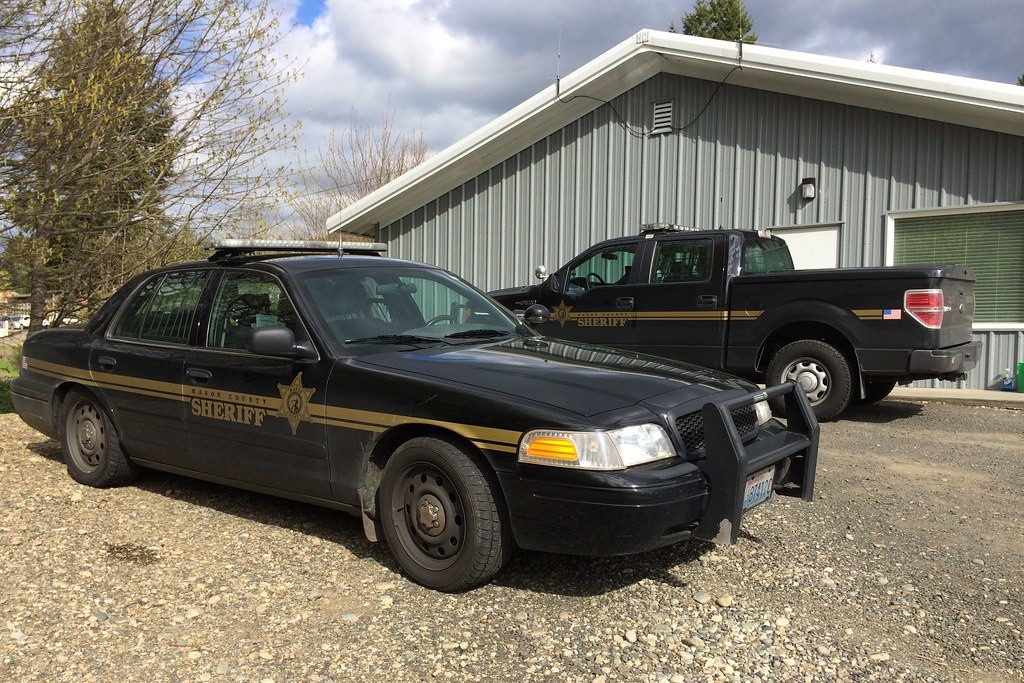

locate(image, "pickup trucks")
(430, 223), (984, 423)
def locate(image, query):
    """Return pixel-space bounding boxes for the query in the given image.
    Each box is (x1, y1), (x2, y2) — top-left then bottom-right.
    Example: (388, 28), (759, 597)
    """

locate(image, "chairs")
(222, 294), (267, 348)
(663, 261), (693, 283)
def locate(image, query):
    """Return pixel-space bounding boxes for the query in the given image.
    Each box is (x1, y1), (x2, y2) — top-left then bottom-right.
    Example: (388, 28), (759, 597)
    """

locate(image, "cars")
(8, 228), (821, 595)
(0, 313), (81, 337)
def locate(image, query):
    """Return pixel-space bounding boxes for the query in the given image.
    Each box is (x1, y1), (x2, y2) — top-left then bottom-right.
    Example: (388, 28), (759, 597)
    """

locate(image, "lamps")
(802, 177), (816, 200)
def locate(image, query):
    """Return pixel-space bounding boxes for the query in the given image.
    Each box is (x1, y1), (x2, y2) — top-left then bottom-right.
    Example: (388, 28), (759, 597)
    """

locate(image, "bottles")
(999, 368), (1017, 391)
